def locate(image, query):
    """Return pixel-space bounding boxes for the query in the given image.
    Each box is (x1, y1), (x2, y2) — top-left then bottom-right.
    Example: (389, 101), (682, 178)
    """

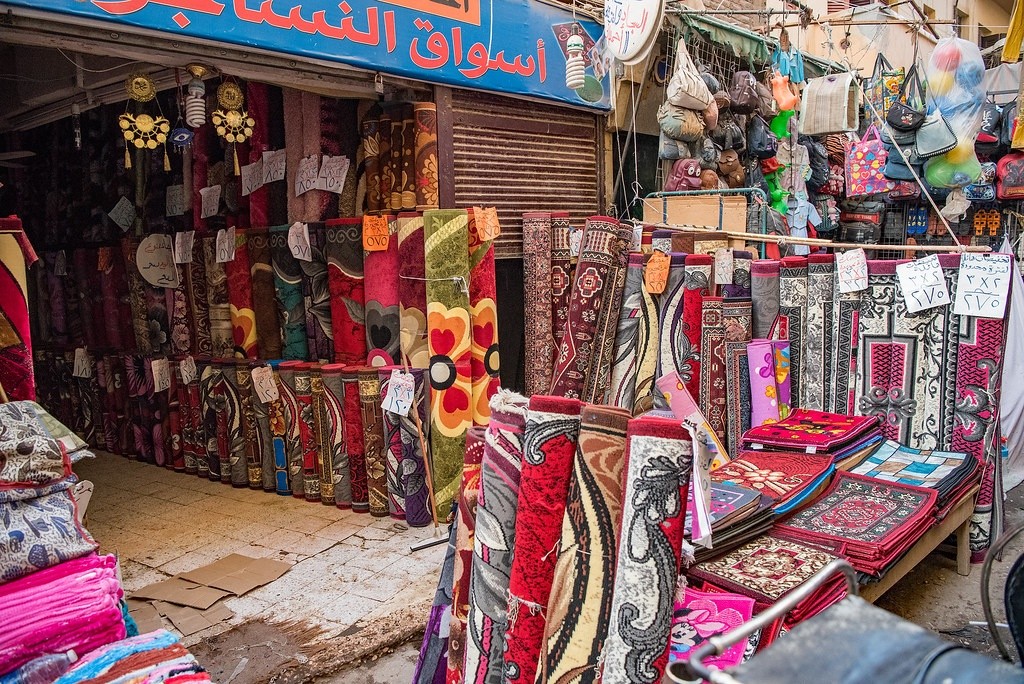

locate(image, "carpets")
(0, 214), (40, 404)
(413, 213), (1015, 684)
(0, 74), (501, 527)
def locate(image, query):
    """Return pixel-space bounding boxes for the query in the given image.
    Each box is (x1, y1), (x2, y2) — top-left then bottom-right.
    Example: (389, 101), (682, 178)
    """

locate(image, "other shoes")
(884, 212), (902, 238)
(907, 206), (1001, 259)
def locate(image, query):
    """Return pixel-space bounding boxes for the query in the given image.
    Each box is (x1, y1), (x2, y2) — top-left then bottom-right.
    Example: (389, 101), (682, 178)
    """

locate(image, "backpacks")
(748, 112), (778, 161)
(729, 69), (758, 115)
(975, 97), (1001, 149)
(996, 152), (1024, 199)
(798, 135), (829, 189)
(1001, 95), (1018, 149)
(964, 161), (997, 202)
(746, 200), (796, 260)
(665, 158), (702, 191)
(756, 81), (778, 122)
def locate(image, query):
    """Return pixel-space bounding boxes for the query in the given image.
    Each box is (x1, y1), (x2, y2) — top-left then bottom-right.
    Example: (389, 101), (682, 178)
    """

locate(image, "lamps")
(185, 63), (209, 128)
(71, 82), (82, 149)
(566, 0), (585, 90)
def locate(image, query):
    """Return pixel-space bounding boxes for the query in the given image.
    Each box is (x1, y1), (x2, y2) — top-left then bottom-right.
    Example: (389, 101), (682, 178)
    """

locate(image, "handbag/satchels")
(667, 38), (713, 110)
(844, 123), (902, 197)
(745, 156), (785, 206)
(658, 129), (691, 160)
(694, 46), (720, 95)
(798, 59), (859, 135)
(862, 53), (905, 134)
(714, 91), (730, 112)
(880, 64), (953, 205)
(656, 98), (706, 142)
(690, 107), (747, 190)
(811, 133), (884, 244)
